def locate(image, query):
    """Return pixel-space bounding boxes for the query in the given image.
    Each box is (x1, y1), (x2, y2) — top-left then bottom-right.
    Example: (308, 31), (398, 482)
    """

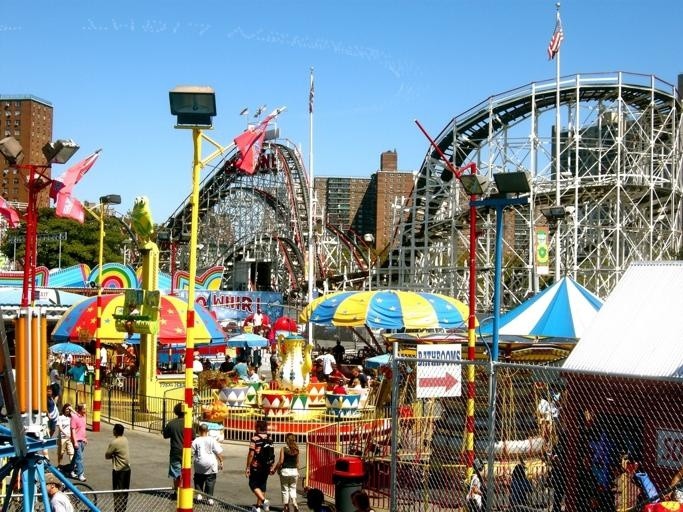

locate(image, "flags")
(233, 113), (277, 175)
(0, 197), (20, 229)
(547, 17), (565, 61)
(55, 193), (85, 224)
(55, 154), (98, 193)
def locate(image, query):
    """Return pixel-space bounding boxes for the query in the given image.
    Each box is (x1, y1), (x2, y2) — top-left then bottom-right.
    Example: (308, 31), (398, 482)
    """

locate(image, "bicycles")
(10, 477), (96, 511)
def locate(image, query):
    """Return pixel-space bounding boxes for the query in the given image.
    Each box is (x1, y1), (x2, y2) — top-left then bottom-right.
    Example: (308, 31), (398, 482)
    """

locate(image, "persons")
(466, 474), (484, 512)
(536, 384), (562, 461)
(90, 281), (104, 291)
(545, 456), (565, 512)
(46, 340), (370, 512)
(253, 309), (263, 334)
(124, 304), (139, 339)
(664, 464), (683, 501)
(510, 464), (537, 512)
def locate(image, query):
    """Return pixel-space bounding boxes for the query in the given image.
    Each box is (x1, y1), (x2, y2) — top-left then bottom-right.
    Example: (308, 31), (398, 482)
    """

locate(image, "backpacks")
(254, 434), (275, 468)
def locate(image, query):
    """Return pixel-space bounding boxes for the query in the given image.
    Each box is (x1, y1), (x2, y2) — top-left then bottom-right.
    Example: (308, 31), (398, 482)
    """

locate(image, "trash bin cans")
(332, 457), (366, 512)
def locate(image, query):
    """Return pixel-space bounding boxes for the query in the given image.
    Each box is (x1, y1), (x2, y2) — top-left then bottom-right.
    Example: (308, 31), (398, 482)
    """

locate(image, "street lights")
(0, 133), (78, 433)
(73, 191), (122, 432)
(165, 84), (219, 512)
(459, 165), (530, 512)
(363, 232), (374, 291)
(537, 198), (575, 283)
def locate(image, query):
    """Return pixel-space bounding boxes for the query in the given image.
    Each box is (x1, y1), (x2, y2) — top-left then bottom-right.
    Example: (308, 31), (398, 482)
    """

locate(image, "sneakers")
(70, 471), (86, 482)
(263, 500), (271, 512)
(251, 507), (260, 512)
(196, 494), (215, 506)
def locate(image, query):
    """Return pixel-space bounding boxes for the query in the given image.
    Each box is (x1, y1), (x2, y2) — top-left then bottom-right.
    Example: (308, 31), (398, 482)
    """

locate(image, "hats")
(44, 473), (61, 486)
